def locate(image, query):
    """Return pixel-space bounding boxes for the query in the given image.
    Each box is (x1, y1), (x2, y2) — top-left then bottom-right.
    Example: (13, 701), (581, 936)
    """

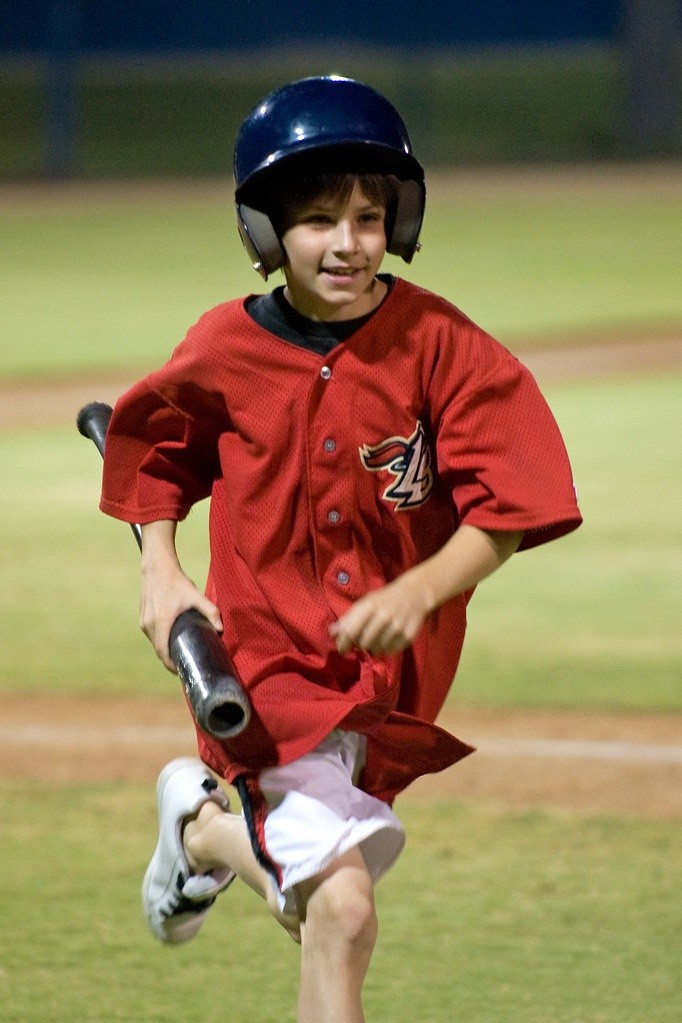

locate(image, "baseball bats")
(75, 399), (253, 737)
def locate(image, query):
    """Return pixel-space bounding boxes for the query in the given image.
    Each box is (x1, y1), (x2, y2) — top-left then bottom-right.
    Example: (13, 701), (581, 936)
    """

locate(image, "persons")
(100, 76), (583, 1023)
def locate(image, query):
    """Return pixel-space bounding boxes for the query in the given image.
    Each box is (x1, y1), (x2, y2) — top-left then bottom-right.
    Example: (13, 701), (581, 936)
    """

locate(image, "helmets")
(233, 75), (427, 282)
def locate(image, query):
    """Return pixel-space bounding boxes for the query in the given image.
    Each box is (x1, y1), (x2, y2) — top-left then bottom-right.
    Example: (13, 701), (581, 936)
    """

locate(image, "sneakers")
(141, 757), (237, 945)
